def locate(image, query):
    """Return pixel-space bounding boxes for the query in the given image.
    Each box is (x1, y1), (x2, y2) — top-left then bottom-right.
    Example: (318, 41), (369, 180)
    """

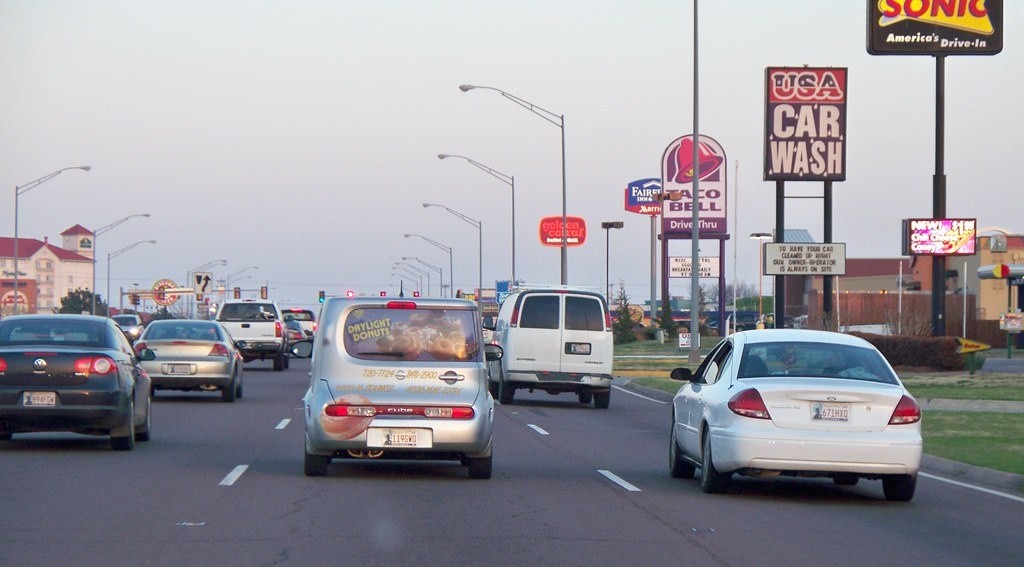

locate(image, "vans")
(482, 285), (613, 409)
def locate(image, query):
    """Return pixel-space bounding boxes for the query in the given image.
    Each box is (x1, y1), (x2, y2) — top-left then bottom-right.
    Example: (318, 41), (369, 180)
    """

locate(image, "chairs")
(744, 354), (768, 377)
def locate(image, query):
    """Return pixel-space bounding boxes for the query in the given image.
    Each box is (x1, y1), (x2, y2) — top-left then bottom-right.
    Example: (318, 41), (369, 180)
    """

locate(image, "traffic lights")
(318, 291), (325, 303)
(346, 291), (354, 297)
(380, 291), (387, 297)
(414, 292), (419, 297)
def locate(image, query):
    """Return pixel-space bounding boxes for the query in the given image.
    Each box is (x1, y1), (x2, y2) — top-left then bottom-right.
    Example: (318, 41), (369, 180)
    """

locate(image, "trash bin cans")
(654, 329), (664, 344)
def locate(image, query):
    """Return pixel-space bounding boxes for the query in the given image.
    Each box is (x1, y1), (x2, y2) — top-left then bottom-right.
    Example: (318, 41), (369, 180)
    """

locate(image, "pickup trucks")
(214, 298), (316, 372)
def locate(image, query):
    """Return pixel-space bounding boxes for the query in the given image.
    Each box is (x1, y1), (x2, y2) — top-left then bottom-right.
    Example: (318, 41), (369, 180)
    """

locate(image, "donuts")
(376, 309), (473, 361)
(321, 394), (373, 440)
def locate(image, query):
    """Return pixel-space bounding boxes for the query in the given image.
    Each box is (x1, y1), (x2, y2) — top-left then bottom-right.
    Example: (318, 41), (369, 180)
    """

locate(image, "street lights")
(107, 240), (156, 319)
(14, 165), (92, 316)
(750, 233), (773, 329)
(390, 233), (453, 299)
(186, 260), (228, 319)
(93, 214), (151, 314)
(601, 221), (623, 304)
(423, 203), (482, 323)
(459, 84), (568, 286)
(437, 153), (515, 287)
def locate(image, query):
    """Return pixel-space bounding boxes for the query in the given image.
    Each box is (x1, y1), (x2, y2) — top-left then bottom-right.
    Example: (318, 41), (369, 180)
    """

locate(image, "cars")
(707, 313), (809, 331)
(109, 314), (243, 403)
(0, 314), (156, 452)
(291, 296), (504, 480)
(669, 328), (924, 501)
